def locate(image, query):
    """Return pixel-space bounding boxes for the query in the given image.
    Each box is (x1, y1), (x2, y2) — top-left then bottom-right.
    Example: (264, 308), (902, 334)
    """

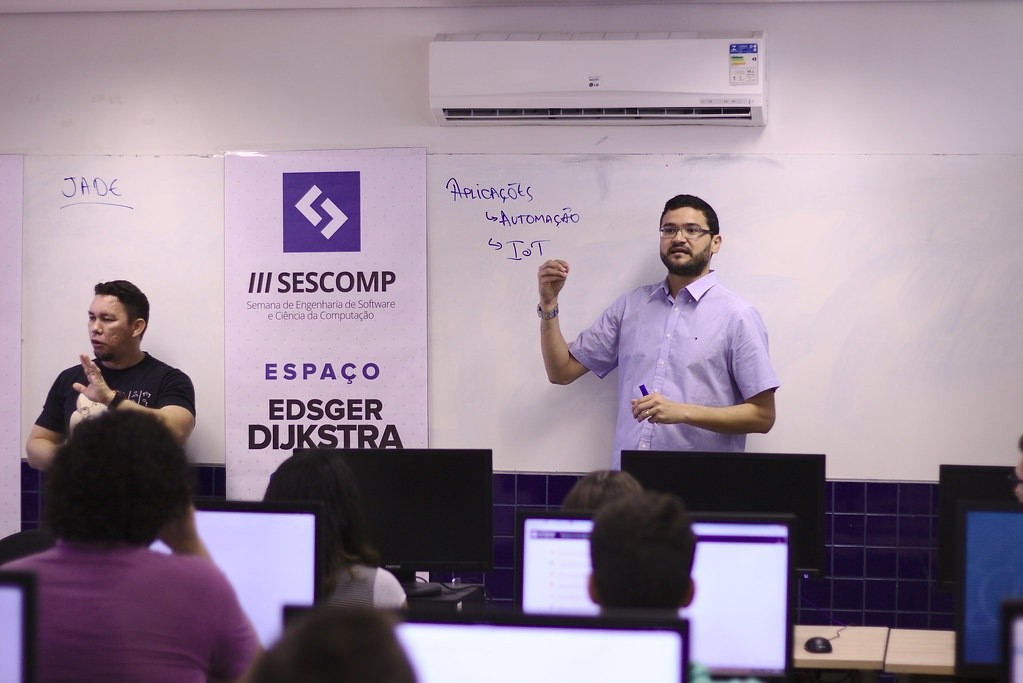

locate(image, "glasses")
(659, 227), (713, 240)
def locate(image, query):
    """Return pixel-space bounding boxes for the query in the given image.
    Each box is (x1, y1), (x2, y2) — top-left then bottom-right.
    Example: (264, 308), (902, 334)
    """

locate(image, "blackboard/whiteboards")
(22, 154), (1023, 486)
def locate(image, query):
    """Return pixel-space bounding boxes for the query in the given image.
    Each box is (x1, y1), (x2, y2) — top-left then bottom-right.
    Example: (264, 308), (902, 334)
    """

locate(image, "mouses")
(805, 636), (832, 653)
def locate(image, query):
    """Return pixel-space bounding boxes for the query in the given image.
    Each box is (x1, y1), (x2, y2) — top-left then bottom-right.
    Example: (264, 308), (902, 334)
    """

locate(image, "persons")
(0, 410), (696, 683)
(23, 280), (195, 470)
(536, 194), (781, 471)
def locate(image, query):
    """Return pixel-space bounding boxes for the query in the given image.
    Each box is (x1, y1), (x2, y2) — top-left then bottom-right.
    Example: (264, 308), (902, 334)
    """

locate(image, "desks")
(792, 623), (957, 683)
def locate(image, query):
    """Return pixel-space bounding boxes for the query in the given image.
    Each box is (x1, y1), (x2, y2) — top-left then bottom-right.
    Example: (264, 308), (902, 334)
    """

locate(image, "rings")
(645, 409), (650, 417)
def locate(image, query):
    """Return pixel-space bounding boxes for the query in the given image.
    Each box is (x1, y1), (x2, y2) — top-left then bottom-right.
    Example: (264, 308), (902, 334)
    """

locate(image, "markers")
(638, 384), (658, 424)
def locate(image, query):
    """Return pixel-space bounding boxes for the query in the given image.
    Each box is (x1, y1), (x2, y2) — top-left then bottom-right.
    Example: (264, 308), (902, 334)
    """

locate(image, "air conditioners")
(428, 31), (768, 128)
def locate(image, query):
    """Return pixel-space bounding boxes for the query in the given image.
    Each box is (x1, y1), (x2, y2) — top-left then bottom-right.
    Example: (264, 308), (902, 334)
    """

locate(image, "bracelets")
(106, 389), (126, 413)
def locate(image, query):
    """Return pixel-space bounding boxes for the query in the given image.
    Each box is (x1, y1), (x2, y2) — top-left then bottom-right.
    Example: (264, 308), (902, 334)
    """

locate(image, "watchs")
(537, 303), (559, 320)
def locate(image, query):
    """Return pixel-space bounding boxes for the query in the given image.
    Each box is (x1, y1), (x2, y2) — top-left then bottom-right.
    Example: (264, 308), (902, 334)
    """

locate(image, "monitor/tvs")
(0, 449), (1023, 682)
